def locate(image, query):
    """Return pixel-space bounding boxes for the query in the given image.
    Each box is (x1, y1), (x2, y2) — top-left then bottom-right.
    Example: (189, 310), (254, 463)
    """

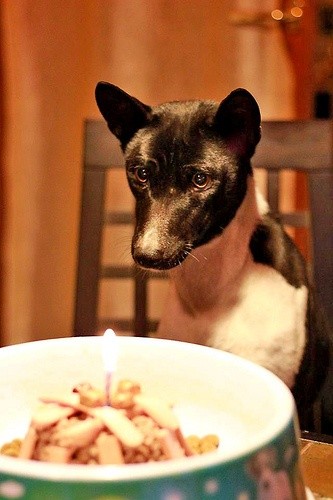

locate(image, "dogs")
(94, 80), (333, 433)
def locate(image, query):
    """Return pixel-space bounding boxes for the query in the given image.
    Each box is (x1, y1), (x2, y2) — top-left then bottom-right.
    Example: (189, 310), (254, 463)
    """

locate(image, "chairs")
(68, 114), (332, 447)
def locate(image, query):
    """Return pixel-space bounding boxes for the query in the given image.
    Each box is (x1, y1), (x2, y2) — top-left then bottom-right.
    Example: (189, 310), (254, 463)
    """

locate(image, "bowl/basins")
(1, 334), (309, 500)
